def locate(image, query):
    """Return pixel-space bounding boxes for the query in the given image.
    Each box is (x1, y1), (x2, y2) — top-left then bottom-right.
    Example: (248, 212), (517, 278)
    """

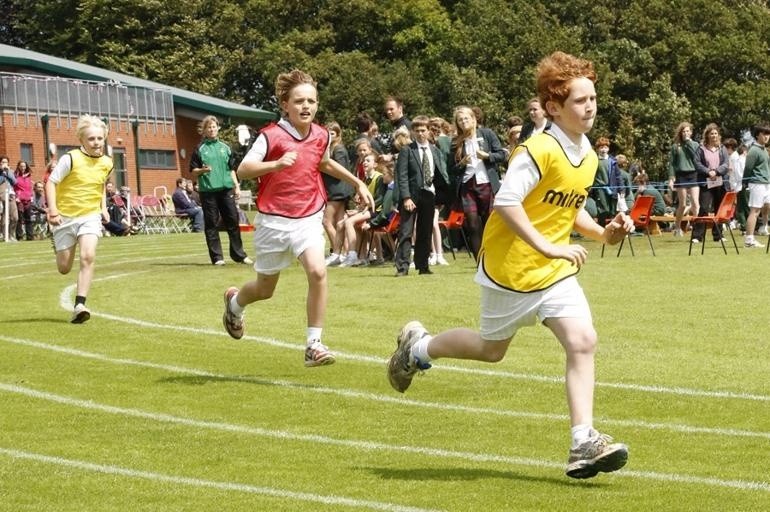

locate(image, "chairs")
(687, 191), (741, 257)
(601, 194), (656, 260)
(355, 210), (399, 265)
(14, 183), (195, 245)
(437, 210), (474, 259)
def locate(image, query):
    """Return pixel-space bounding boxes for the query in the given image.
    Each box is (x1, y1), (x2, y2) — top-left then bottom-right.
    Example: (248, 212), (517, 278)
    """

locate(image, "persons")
(189, 114), (254, 267)
(324, 97), (548, 277)
(385, 50), (637, 478)
(1, 158), (58, 242)
(221, 64), (378, 368)
(171, 178), (208, 231)
(43, 112), (116, 325)
(572, 119), (770, 249)
(105, 181), (145, 238)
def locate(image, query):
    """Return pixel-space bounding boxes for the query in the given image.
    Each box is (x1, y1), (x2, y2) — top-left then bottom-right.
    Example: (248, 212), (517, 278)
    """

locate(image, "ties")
(421, 147), (432, 186)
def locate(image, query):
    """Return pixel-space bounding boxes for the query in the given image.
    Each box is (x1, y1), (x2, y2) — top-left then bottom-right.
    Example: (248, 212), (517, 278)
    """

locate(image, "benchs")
(646, 211), (677, 236)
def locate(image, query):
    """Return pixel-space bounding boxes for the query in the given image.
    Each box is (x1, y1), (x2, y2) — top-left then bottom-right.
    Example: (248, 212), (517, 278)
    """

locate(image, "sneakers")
(566, 428), (628, 478)
(743, 239), (766, 248)
(223, 287), (244, 339)
(305, 339), (336, 366)
(325, 250), (448, 276)
(386, 321), (432, 392)
(71, 303), (90, 323)
